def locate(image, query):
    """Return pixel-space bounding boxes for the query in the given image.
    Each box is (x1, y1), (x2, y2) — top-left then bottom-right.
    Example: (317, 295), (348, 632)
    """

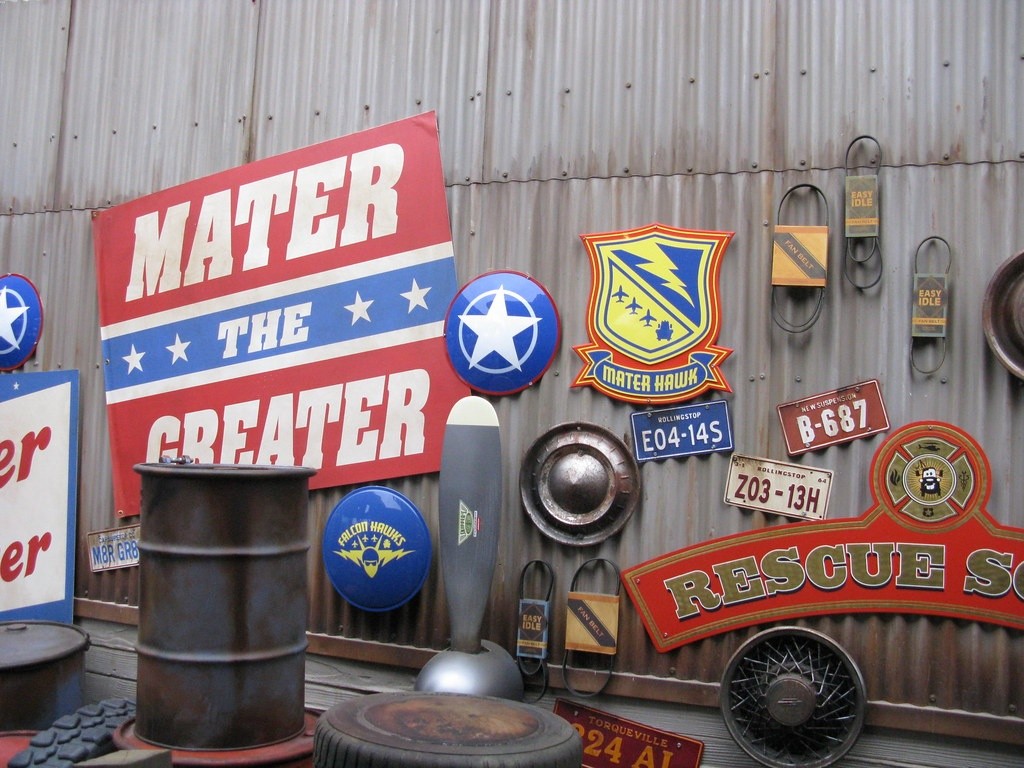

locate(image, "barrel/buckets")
(131, 462), (316, 752)
(0, 730), (39, 768)
(0, 620), (91, 730)
(112, 707), (324, 768)
